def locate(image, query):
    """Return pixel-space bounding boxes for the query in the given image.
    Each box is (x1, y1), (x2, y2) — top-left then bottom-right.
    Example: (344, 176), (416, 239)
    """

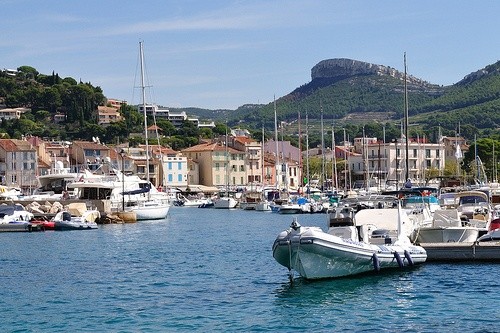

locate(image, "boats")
(272, 194), (427, 282)
(0, 39), (500, 261)
